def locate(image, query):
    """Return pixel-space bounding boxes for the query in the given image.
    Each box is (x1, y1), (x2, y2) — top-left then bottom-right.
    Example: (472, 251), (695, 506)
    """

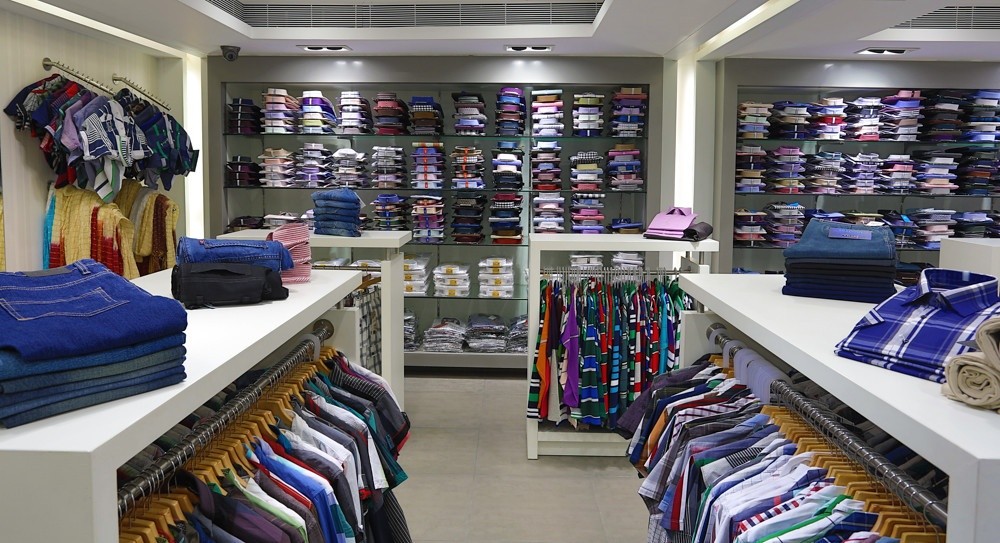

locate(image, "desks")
(939, 238), (1000, 279)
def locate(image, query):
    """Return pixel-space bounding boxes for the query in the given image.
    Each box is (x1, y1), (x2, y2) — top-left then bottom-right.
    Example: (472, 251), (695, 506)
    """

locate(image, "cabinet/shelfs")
(528, 233), (720, 458)
(680, 274), (1000, 543)
(220, 81), (650, 368)
(0, 265), (361, 543)
(733, 84), (1000, 252)
(216, 229), (413, 412)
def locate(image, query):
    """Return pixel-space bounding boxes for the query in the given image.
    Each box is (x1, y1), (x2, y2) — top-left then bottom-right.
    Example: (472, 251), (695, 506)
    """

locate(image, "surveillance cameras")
(221, 46), (240, 62)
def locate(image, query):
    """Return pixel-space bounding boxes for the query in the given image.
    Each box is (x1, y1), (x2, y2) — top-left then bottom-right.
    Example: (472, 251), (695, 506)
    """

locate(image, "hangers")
(542, 265), (948, 543)
(117, 266), (390, 543)
(25, 64), (188, 137)
